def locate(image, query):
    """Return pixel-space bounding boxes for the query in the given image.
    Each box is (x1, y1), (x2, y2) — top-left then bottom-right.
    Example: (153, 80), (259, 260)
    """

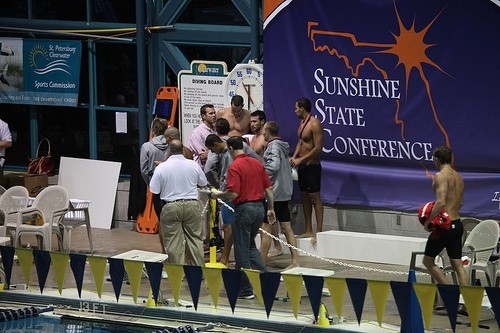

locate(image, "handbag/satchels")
(27, 137), (55, 177)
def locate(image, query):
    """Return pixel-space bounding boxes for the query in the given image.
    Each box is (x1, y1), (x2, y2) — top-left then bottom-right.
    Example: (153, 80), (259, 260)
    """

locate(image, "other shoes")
(237, 289), (254, 299)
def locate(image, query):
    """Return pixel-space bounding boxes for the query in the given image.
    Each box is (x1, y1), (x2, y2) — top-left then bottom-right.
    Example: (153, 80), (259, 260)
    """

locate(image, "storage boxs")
(12, 173), (49, 197)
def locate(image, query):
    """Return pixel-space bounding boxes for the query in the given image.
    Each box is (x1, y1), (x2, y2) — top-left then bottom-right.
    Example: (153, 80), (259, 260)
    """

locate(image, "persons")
(215, 95), (253, 136)
(205, 134), (234, 267)
(289, 98), (324, 239)
(424, 147), (470, 289)
(140, 118), (171, 219)
(261, 122), (299, 272)
(247, 111), (284, 256)
(188, 104), (220, 263)
(211, 136), (285, 300)
(203, 117), (266, 190)
(154, 127), (185, 167)
(0, 119), (12, 174)
(150, 139), (208, 280)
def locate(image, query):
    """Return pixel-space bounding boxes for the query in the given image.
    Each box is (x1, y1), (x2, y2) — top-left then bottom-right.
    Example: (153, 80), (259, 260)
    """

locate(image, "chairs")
(408, 217), (500, 315)
(0, 185), (94, 265)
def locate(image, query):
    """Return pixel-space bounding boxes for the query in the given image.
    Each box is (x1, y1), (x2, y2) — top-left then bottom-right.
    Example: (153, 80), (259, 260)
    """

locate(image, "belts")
(167, 198), (198, 203)
